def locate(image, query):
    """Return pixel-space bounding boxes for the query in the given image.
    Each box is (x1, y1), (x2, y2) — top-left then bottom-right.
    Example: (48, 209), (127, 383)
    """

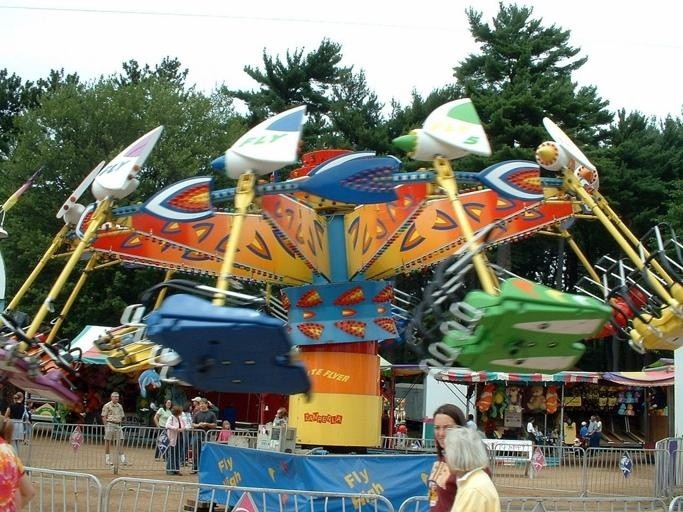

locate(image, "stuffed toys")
(476, 381), (560, 419)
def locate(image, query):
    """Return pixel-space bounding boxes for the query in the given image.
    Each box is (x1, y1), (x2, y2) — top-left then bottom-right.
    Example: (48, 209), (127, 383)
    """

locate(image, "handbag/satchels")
(154, 428), (171, 456)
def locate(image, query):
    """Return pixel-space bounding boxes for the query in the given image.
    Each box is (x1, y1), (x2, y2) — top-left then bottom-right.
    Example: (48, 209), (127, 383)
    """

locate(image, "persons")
(444, 426), (501, 512)
(272, 407), (288, 427)
(430, 404), (492, 512)
(527, 415), (603, 457)
(467, 414), (477, 428)
(0, 391), (35, 512)
(149, 396), (237, 475)
(100, 391), (134, 466)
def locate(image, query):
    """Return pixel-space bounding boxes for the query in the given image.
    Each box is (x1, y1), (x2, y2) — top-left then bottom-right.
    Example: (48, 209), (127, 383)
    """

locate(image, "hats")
(581, 421), (587, 426)
(190, 396), (201, 403)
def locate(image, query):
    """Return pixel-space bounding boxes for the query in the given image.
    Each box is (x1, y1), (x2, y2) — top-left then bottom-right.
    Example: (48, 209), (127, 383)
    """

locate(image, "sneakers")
(106, 457), (197, 476)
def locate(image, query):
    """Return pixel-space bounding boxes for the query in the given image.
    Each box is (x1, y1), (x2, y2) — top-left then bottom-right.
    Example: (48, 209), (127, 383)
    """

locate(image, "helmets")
(397, 424), (408, 433)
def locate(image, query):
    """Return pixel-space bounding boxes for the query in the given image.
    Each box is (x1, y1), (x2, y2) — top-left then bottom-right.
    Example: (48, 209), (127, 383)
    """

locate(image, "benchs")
(482, 439), (533, 479)
